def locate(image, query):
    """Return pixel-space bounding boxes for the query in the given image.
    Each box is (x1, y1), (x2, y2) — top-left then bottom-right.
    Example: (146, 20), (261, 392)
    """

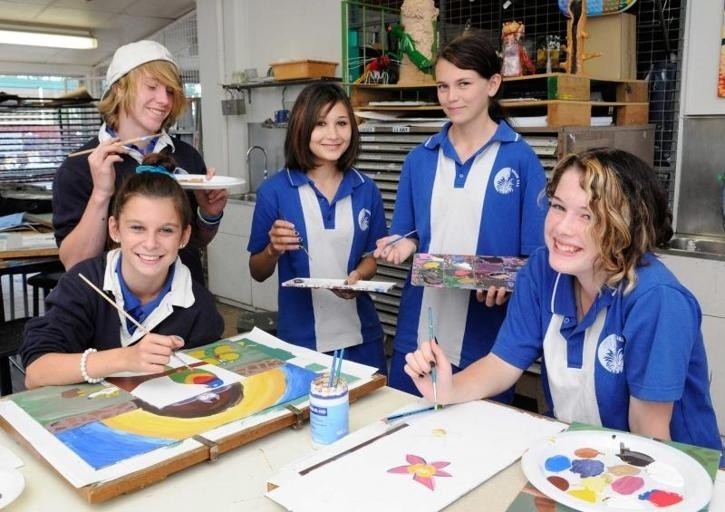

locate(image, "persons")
(50, 39), (226, 293)
(247, 80), (390, 386)
(404, 148), (724, 471)
(19, 168), (226, 386)
(373, 36), (551, 407)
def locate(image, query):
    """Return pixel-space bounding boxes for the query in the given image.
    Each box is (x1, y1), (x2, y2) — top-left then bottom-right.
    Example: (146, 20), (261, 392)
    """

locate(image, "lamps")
(0, 30), (100, 52)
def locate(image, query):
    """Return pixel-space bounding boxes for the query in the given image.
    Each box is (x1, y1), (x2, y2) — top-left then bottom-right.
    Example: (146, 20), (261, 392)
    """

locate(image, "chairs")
(27, 272), (64, 317)
(0, 257), (64, 399)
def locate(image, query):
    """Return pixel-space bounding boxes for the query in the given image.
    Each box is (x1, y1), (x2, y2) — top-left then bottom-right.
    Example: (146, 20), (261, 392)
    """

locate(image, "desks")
(0, 385), (725, 512)
(0, 229), (60, 261)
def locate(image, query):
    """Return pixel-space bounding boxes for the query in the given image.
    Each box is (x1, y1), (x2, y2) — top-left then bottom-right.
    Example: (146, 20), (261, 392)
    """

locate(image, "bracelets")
(79, 346), (107, 384)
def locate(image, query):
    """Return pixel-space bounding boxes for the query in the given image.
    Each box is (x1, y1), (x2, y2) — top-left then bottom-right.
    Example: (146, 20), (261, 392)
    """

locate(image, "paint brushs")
(300, 243), (312, 260)
(361, 229), (419, 257)
(328, 350), (338, 386)
(334, 348), (347, 388)
(77, 272), (196, 374)
(427, 306), (437, 411)
(67, 133), (164, 157)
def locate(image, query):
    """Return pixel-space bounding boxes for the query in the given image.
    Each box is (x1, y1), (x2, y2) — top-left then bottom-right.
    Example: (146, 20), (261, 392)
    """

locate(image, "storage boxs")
(567, 12), (637, 81)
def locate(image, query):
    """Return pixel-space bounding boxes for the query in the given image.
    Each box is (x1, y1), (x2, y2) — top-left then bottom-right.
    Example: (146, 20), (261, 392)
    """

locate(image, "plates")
(520, 430), (714, 512)
(175, 174), (248, 195)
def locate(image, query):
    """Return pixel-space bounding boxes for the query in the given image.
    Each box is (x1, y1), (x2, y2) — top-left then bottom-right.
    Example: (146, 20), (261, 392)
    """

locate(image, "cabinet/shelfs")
(349, 71), (657, 417)
(653, 253), (725, 447)
(207, 200), (279, 313)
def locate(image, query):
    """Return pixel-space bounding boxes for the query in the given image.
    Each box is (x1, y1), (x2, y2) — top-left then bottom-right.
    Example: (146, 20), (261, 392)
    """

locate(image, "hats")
(101, 39), (178, 104)
